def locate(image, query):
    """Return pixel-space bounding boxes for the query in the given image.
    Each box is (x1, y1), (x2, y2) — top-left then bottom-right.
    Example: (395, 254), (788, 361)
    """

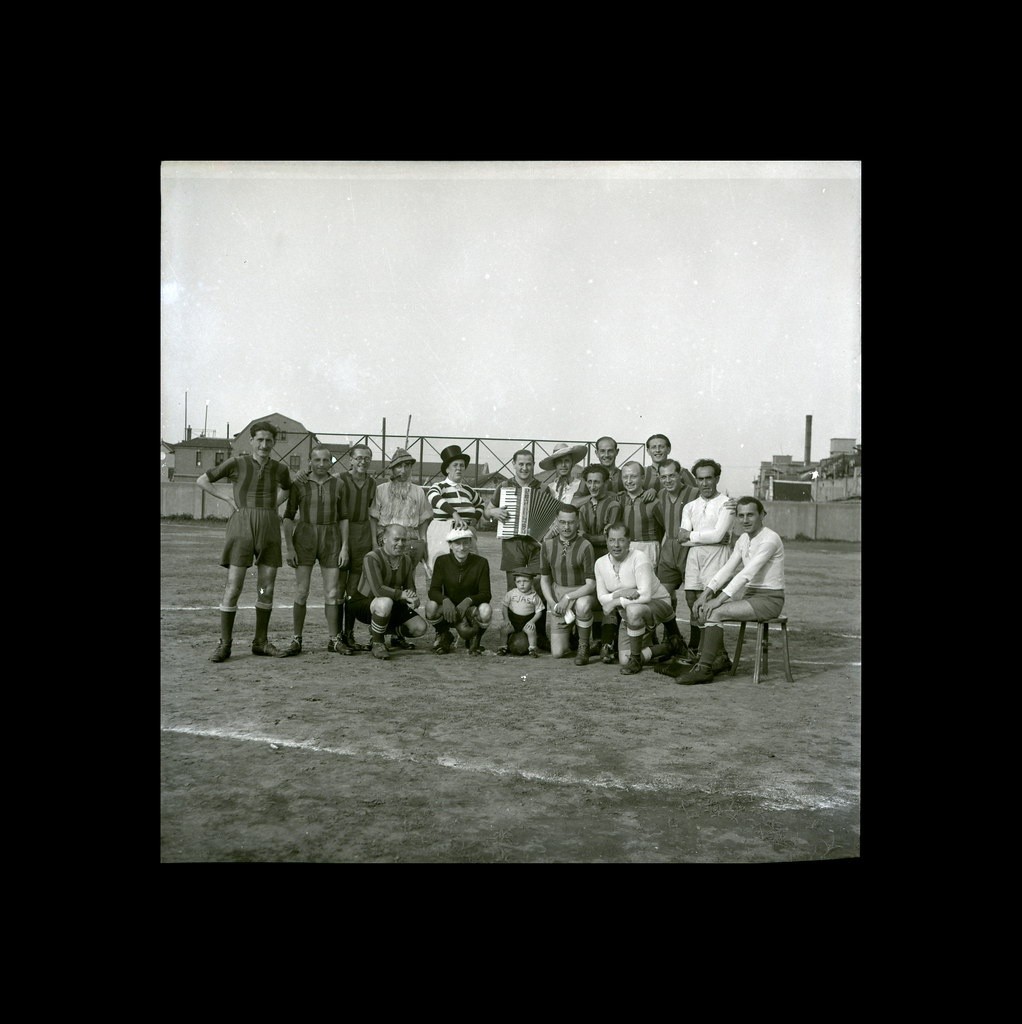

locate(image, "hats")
(389, 447), (416, 468)
(539, 443), (588, 470)
(512, 567), (537, 578)
(446, 527), (473, 541)
(441, 445), (471, 476)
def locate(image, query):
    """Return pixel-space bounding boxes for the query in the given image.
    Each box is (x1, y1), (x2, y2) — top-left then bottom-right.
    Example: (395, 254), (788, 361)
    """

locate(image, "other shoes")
(465, 640), (485, 652)
(620, 636), (733, 685)
(344, 631), (361, 649)
(575, 639), (591, 665)
(289, 636), (302, 655)
(529, 651), (538, 658)
(391, 637), (416, 650)
(210, 638), (233, 661)
(469, 634), (481, 656)
(363, 636), (373, 650)
(372, 642), (391, 660)
(328, 633), (354, 655)
(252, 640), (288, 658)
(601, 640), (615, 664)
(536, 635), (551, 652)
(589, 639), (600, 655)
(434, 632), (454, 654)
(497, 647), (508, 656)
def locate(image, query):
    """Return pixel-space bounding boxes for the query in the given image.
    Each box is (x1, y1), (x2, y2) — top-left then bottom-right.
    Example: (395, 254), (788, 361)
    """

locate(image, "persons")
(496, 565), (546, 659)
(569, 437), (658, 662)
(282, 443), (354, 657)
(482, 448), (554, 652)
(349, 523), (429, 661)
(642, 434), (698, 492)
(593, 523), (687, 676)
(677, 459), (735, 661)
(613, 459), (738, 659)
(367, 447), (434, 651)
(295, 443), (377, 653)
(423, 525), (493, 655)
(195, 420), (293, 663)
(676, 495), (787, 686)
(540, 504), (599, 666)
(537, 440), (589, 649)
(426, 444), (487, 649)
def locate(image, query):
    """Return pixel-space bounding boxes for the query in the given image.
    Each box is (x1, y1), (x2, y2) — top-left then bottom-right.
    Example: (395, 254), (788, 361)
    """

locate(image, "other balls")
(508, 632), (529, 655)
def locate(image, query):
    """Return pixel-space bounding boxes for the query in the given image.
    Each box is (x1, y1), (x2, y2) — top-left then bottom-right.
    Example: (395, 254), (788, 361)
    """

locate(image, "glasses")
(351, 457), (371, 462)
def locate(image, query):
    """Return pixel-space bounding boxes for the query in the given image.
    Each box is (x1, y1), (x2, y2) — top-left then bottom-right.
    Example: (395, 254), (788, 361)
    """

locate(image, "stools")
(732, 618), (794, 685)
